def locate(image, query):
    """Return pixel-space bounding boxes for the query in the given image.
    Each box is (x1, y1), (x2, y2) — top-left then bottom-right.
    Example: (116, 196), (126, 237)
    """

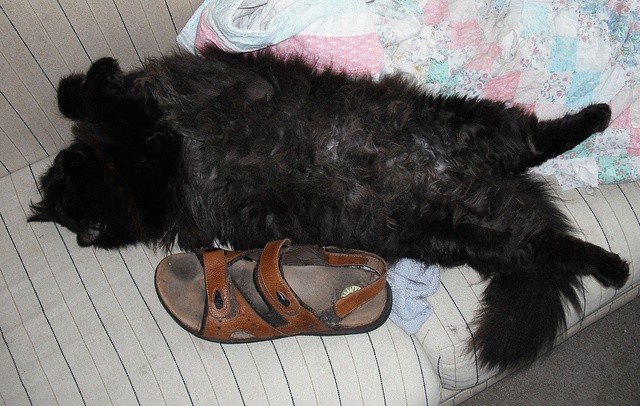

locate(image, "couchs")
(0, 0), (640, 405)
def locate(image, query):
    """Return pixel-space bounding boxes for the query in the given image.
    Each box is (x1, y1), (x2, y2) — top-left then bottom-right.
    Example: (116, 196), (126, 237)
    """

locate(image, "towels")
(347, 257), (440, 335)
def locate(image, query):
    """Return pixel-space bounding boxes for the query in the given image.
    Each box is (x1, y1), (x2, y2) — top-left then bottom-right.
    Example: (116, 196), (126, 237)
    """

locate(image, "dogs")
(26, 38), (633, 377)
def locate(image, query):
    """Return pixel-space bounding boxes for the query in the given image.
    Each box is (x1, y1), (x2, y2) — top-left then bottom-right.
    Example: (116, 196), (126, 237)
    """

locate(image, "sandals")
(154, 238), (394, 344)
